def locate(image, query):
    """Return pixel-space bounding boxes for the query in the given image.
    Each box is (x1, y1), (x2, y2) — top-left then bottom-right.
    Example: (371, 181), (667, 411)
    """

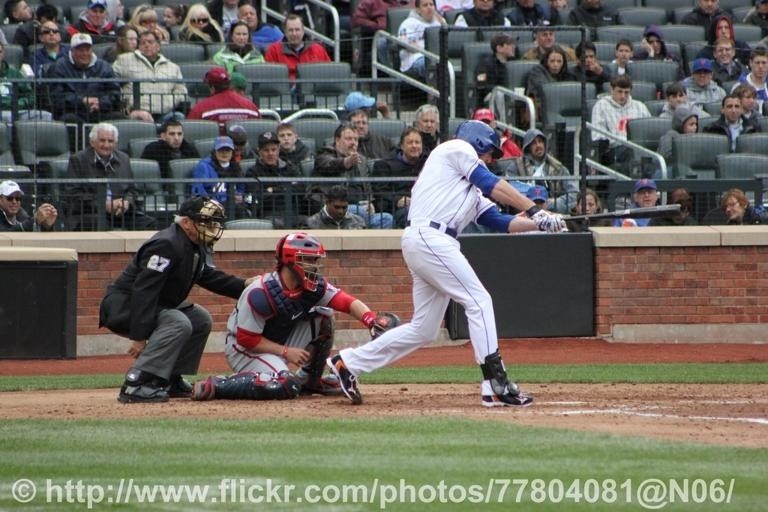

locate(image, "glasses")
(191, 18), (208, 24)
(42, 29), (59, 33)
(231, 20), (248, 25)
(4, 196), (21, 201)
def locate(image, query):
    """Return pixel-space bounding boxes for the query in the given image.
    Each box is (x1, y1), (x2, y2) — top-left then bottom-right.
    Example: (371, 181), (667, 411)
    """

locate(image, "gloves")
(527, 208), (570, 232)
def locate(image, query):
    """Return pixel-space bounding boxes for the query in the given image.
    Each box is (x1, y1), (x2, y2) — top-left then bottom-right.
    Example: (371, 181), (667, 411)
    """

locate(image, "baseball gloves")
(370, 311), (401, 338)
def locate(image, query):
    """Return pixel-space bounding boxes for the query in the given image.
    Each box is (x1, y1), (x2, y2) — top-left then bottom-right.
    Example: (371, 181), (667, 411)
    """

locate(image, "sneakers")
(295, 369), (342, 396)
(482, 393), (535, 407)
(118, 383), (169, 402)
(195, 379), (213, 399)
(327, 355), (362, 404)
(158, 374), (192, 398)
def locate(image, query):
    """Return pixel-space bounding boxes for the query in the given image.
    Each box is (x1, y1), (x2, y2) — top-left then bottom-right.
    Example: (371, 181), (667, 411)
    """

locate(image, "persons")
(326, 118), (567, 407)
(191, 230), (402, 402)
(0, 0), (768, 228)
(99, 195), (263, 403)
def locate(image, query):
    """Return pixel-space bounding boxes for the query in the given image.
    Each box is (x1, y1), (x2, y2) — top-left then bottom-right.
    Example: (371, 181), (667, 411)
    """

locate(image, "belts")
(404, 220), (457, 238)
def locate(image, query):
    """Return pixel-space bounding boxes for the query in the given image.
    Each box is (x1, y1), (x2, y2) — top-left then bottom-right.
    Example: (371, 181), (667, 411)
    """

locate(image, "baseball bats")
(538, 203), (680, 231)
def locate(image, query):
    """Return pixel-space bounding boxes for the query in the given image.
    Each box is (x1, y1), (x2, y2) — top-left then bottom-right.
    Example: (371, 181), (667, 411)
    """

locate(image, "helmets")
(276, 234), (326, 292)
(179, 196), (226, 253)
(455, 120), (503, 159)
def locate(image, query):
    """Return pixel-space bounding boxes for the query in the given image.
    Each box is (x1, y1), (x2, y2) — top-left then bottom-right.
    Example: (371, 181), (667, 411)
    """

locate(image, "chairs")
(0, 0), (768, 230)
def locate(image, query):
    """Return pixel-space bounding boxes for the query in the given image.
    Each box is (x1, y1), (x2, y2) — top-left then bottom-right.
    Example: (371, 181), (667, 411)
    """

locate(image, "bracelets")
(282, 343), (289, 359)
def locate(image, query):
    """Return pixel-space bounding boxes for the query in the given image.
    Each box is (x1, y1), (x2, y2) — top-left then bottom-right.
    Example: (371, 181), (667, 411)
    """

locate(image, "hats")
(491, 33), (519, 48)
(474, 110), (495, 122)
(693, 58), (714, 74)
(0, 180), (25, 196)
(214, 136), (235, 151)
(228, 72), (246, 89)
(533, 20), (554, 41)
(634, 179), (658, 193)
(88, 0), (107, 10)
(71, 33), (93, 47)
(344, 92), (375, 112)
(526, 186), (549, 203)
(206, 69), (231, 85)
(259, 132), (280, 147)
